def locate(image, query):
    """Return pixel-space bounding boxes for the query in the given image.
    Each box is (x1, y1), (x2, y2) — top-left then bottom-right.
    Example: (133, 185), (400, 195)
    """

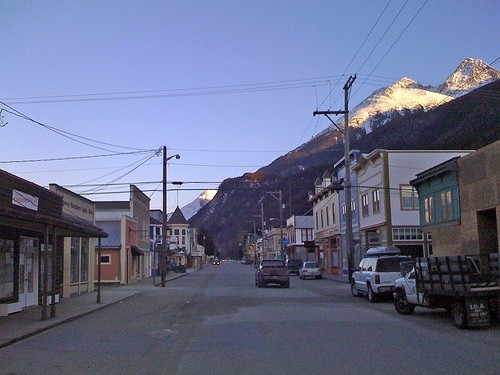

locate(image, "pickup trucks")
(392, 256), (500, 329)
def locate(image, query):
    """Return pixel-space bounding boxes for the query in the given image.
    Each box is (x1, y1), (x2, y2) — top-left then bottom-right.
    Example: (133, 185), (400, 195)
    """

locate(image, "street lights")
(160, 153), (180, 287)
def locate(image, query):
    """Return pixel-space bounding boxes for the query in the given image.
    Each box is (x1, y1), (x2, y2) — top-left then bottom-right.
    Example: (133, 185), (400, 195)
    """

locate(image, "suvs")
(350, 247), (414, 303)
(255, 259), (290, 288)
(286, 258), (303, 275)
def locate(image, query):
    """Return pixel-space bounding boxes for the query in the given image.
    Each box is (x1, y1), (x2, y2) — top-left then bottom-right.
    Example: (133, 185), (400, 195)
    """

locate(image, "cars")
(212, 260), (220, 265)
(299, 262), (322, 279)
